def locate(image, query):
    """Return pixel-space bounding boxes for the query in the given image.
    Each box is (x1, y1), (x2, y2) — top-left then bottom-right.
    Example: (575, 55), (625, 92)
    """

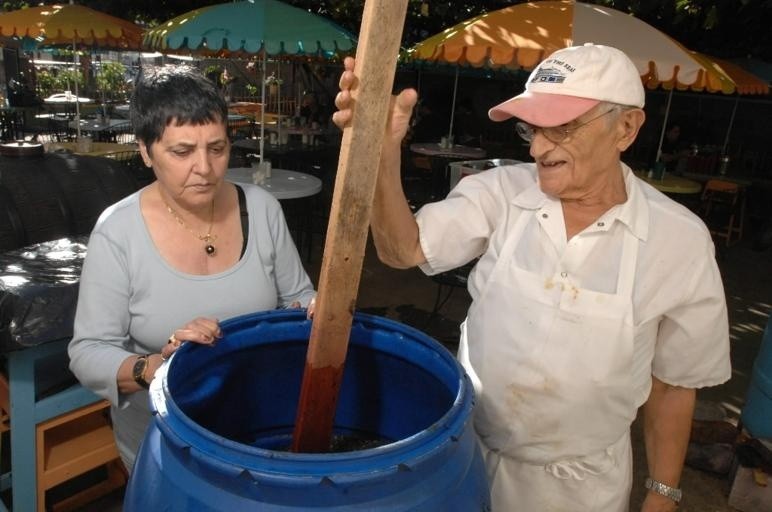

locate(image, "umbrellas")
(140, 1), (357, 160)
(0, 1), (144, 135)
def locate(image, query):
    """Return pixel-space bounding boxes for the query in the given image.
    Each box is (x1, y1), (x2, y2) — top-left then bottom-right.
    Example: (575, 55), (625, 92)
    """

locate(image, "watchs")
(132, 353), (155, 391)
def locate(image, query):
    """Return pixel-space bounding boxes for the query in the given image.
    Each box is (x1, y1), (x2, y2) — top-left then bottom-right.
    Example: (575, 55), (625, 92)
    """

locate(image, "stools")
(0, 337), (129, 512)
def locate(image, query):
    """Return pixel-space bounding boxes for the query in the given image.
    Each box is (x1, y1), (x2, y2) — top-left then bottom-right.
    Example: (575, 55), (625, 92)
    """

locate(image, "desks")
(0, 96), (752, 262)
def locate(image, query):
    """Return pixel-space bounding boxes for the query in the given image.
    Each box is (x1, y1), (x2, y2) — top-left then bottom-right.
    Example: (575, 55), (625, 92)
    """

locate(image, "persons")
(332, 41), (735, 512)
(65, 71), (318, 483)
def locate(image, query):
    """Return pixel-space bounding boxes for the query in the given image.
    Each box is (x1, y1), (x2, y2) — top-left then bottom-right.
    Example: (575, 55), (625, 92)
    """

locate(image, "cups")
(64, 90), (72, 101)
(270, 133), (277, 145)
(251, 159), (272, 185)
(281, 130), (288, 144)
(652, 162), (666, 182)
(17, 139), (24, 147)
(286, 118), (292, 129)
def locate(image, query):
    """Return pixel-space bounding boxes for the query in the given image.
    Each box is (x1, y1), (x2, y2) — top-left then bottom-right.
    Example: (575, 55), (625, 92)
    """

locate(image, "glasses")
(517, 106), (618, 145)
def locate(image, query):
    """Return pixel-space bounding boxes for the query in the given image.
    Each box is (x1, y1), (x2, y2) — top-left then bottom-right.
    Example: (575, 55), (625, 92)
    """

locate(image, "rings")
(166, 333), (176, 345)
(159, 353), (168, 361)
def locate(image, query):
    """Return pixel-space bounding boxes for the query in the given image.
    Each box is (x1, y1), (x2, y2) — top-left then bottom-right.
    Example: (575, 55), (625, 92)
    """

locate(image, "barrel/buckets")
(739, 326), (771, 439)
(121, 309), (490, 511)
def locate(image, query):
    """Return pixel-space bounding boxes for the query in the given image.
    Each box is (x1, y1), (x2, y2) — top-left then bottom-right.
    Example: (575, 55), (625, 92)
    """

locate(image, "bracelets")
(644, 476), (684, 507)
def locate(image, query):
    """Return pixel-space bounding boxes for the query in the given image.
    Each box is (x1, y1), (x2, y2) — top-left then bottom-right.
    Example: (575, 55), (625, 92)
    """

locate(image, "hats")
(489, 42), (647, 128)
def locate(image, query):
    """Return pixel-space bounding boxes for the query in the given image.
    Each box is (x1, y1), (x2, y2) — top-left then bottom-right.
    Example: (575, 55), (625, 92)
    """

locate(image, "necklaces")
(159, 183), (219, 260)
